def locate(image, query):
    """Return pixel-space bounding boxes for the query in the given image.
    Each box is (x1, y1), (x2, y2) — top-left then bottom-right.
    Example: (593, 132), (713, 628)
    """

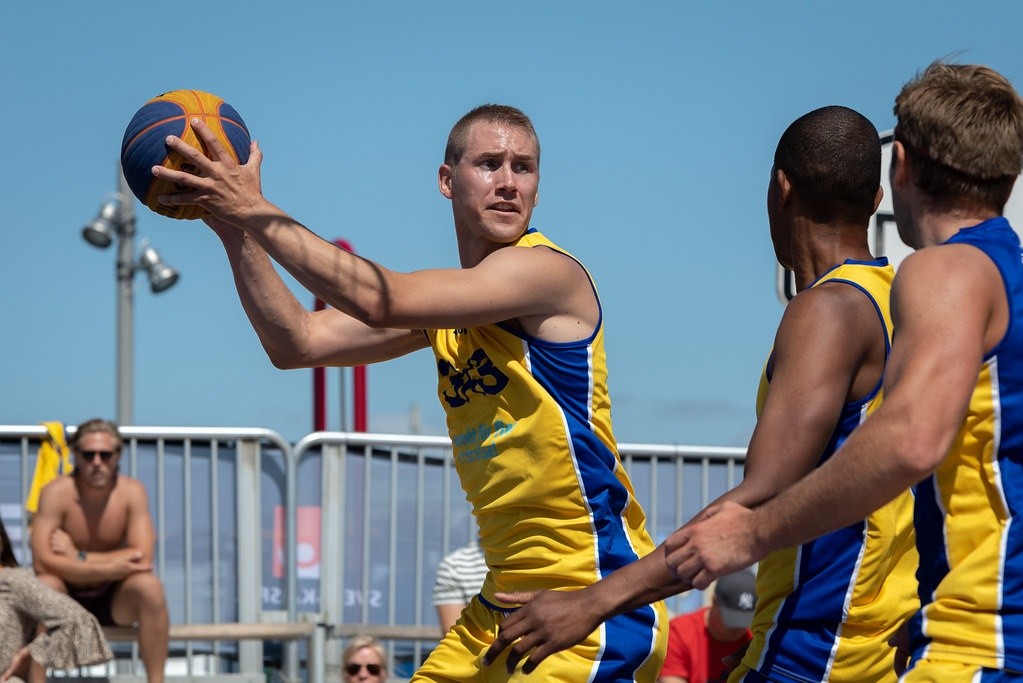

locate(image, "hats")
(715, 570), (756, 627)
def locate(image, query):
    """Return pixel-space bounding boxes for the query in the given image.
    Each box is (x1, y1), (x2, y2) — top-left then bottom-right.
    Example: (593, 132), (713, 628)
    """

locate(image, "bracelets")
(78, 551), (85, 561)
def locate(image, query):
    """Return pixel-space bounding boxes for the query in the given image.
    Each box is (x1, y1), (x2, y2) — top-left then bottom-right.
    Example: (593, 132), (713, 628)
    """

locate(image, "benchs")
(103, 620), (441, 683)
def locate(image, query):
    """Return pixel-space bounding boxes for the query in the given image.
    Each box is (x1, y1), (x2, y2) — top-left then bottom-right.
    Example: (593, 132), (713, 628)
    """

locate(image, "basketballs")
(120, 89), (251, 221)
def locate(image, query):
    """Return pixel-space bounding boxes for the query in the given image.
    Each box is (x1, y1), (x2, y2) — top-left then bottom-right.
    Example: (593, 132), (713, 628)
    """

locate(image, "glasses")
(345, 663), (383, 676)
(78, 448), (117, 461)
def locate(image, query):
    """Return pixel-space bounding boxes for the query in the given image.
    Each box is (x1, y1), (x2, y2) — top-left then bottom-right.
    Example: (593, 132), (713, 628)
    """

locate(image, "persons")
(655, 566), (757, 683)
(0, 521), (116, 683)
(662, 63), (1023, 683)
(479, 105), (919, 683)
(32, 420), (169, 683)
(152, 104), (669, 683)
(433, 539), (489, 637)
(341, 635), (388, 683)
